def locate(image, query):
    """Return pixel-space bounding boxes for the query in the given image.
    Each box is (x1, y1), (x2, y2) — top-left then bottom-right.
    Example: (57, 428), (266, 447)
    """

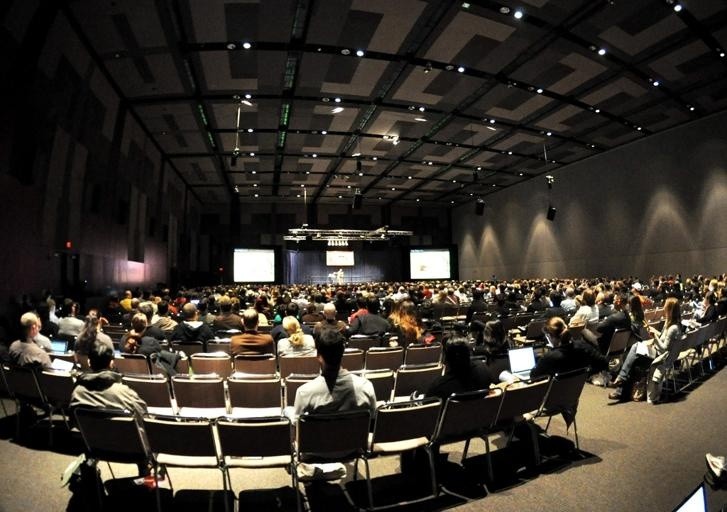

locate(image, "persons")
(7, 268), (726, 472)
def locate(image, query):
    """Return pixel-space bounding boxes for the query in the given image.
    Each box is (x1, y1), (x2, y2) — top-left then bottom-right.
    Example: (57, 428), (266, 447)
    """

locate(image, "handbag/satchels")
(631, 376), (648, 402)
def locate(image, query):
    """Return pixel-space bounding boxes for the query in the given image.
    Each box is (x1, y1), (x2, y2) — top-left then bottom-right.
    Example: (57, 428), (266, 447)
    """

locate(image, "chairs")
(351, 396), (444, 512)
(425, 385), (505, 499)
(66, 401), (173, 512)
(215, 414), (303, 512)
(507, 365), (591, 466)
(2, 283), (726, 439)
(285, 406), (374, 512)
(139, 413), (231, 511)
(460, 375), (551, 484)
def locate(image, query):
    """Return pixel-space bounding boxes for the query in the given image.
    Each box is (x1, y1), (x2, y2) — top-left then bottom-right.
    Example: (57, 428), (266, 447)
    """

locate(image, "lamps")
(327, 240), (349, 247)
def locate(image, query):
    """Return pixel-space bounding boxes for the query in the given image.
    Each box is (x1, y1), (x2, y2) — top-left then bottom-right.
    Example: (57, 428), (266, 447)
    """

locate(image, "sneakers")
(705, 453), (724, 477)
(607, 377), (626, 388)
(608, 398), (623, 405)
(609, 388), (623, 399)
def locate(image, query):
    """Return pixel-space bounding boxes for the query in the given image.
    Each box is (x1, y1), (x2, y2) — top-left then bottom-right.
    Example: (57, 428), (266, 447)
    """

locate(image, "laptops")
(45, 341), (68, 355)
(508, 347), (535, 381)
(672, 481), (707, 512)
